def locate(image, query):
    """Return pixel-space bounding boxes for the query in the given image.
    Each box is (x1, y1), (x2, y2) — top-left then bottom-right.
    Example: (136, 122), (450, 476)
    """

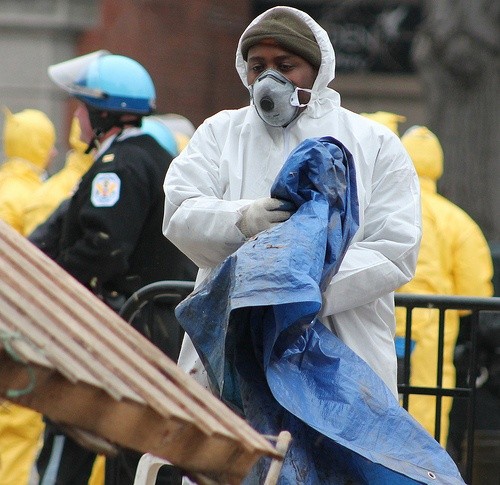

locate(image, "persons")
(25, 49), (198, 485)
(394, 126), (494, 450)
(0, 110), (104, 485)
(162, 5), (420, 401)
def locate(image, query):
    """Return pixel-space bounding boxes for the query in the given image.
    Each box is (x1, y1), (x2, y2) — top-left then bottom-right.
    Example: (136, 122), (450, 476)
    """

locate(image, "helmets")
(47, 48), (157, 129)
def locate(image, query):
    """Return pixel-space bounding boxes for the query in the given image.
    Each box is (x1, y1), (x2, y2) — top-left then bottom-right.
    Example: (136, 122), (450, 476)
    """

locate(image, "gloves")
(237, 198), (294, 239)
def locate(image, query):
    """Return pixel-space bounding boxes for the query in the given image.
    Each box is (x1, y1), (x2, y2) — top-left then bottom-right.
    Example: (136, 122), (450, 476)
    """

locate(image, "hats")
(240, 7), (322, 70)
